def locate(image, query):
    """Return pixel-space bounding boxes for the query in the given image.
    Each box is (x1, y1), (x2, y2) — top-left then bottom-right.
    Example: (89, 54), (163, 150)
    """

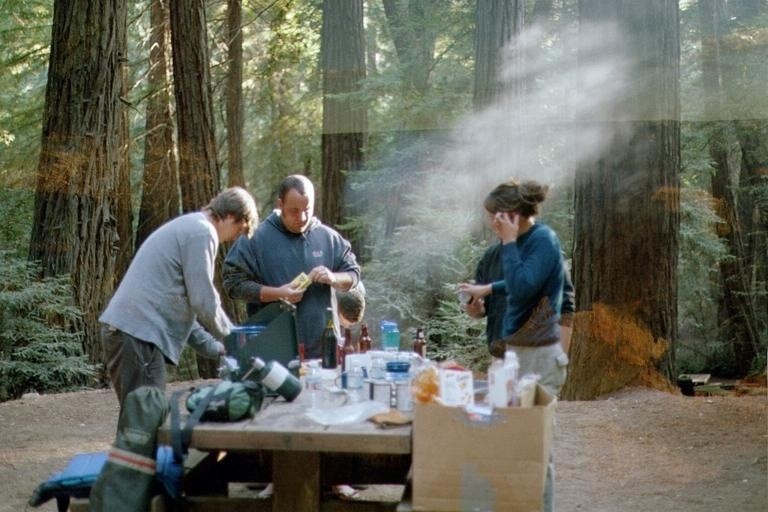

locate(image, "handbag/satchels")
(172, 378), (263, 462)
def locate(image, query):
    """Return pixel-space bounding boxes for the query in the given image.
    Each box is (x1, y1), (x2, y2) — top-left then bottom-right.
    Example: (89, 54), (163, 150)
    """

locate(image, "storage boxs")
(411, 380), (558, 512)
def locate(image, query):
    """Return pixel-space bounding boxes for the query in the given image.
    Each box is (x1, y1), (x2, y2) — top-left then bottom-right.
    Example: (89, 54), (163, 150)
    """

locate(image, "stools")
(28, 445), (183, 511)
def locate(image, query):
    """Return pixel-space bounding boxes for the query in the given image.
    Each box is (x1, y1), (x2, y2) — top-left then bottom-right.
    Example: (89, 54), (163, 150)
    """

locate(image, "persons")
(223, 175), (360, 501)
(461, 237), (507, 364)
(454, 180), (568, 512)
(90, 187), (259, 408)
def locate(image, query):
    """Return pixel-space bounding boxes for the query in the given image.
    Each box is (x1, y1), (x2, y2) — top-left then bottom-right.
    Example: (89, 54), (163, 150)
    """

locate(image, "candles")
(298, 342), (306, 363)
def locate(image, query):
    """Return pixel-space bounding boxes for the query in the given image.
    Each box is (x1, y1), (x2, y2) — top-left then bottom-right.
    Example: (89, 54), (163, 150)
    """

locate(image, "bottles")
(346, 360), (365, 405)
(384, 362), (412, 409)
(249, 356), (302, 402)
(357, 323), (372, 354)
(413, 327), (426, 361)
(320, 308), (339, 369)
(503, 351), (518, 406)
(341, 327), (354, 390)
(487, 358), (507, 410)
(369, 355), (386, 380)
(381, 321), (400, 350)
